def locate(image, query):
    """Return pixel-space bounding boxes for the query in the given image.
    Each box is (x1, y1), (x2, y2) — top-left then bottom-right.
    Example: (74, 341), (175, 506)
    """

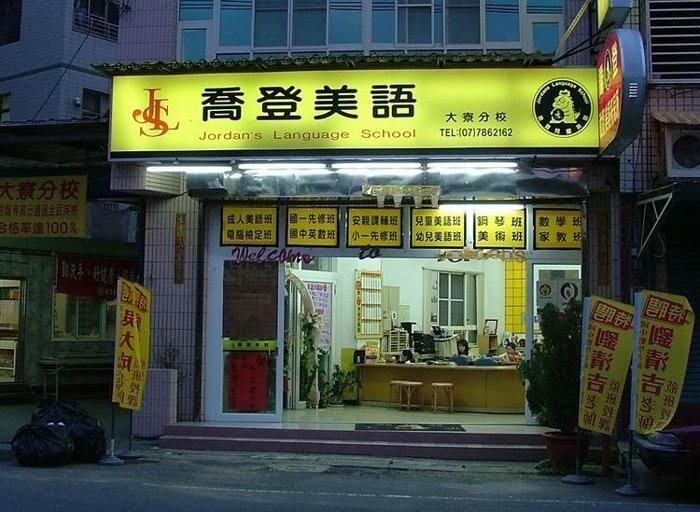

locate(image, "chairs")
(474, 358), (497, 366)
(448, 356), (470, 366)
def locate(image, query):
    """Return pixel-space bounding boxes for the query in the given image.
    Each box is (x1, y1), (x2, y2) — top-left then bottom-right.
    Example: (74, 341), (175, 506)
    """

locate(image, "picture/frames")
(484, 318), (498, 335)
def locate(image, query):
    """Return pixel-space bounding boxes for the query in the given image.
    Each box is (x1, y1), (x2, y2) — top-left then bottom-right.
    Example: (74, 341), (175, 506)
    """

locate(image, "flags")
(579, 295), (635, 435)
(629, 290), (695, 434)
(111, 278), (150, 411)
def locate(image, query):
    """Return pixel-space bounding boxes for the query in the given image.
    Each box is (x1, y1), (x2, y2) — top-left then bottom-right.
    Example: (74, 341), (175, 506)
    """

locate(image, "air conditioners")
(665, 124), (700, 182)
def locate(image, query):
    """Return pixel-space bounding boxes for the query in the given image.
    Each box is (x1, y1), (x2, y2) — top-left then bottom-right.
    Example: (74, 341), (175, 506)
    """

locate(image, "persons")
(491, 342), (521, 363)
(454, 339), (473, 359)
(402, 349), (416, 364)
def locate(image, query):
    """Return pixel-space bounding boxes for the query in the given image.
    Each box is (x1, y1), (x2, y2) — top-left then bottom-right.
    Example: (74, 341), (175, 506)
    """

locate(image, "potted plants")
(326, 365), (362, 404)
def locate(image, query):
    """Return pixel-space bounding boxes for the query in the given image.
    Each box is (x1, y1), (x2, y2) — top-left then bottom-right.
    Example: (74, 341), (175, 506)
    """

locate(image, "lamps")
(229, 161), (243, 180)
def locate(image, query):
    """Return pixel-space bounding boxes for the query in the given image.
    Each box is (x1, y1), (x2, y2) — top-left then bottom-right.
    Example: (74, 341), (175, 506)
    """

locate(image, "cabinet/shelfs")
(383, 329), (407, 354)
(478, 334), (498, 357)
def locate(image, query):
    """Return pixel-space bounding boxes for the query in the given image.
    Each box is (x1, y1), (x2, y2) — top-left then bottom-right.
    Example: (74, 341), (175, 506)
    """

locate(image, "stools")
(398, 381), (426, 412)
(388, 379), (410, 411)
(431, 382), (456, 413)
